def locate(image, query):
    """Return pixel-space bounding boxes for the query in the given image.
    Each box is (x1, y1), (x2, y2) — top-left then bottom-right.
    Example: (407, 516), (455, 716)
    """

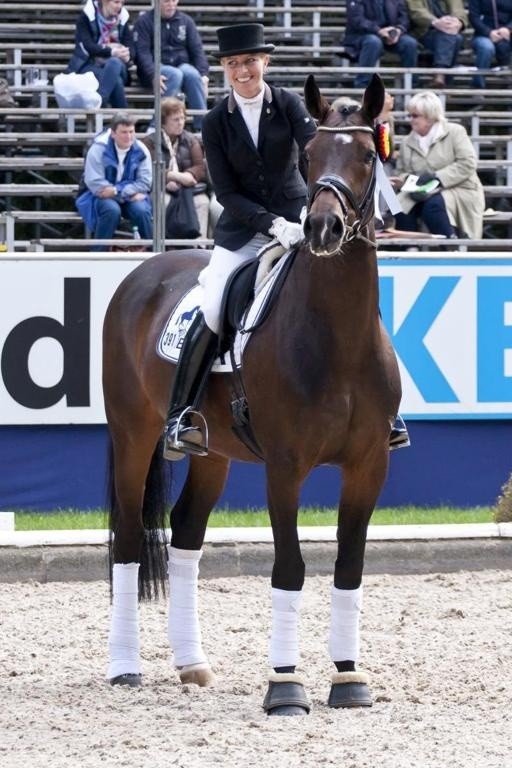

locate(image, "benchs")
(1, 0), (511, 251)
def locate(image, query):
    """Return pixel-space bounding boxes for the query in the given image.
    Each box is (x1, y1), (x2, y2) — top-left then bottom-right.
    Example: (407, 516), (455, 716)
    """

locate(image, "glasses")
(405, 111), (424, 120)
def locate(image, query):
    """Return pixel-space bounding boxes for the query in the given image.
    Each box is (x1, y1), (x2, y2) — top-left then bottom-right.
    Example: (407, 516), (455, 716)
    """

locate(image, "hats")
(211, 22), (275, 58)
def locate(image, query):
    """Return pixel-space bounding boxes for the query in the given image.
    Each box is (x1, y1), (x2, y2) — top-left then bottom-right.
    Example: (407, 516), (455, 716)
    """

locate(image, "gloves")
(268, 216), (307, 251)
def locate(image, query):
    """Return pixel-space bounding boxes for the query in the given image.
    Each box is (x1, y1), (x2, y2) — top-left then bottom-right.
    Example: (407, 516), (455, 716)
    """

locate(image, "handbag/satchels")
(165, 183), (203, 239)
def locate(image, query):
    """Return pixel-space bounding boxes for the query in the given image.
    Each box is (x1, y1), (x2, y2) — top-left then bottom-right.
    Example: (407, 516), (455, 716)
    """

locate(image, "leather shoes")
(388, 426), (408, 449)
(161, 417), (204, 462)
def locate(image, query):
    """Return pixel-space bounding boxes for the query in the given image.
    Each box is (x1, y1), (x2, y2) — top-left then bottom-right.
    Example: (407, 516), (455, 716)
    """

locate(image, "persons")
(74, 97), (154, 252)
(162, 25), (412, 462)
(141, 96), (209, 250)
(343, 0), (512, 87)
(391, 89), (486, 253)
(134, 0), (210, 133)
(63, 0), (137, 110)
(373, 92), (398, 233)
(203, 152), (225, 229)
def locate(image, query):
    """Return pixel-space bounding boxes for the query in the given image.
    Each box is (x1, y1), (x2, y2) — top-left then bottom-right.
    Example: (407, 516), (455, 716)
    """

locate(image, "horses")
(102, 72), (402, 716)
